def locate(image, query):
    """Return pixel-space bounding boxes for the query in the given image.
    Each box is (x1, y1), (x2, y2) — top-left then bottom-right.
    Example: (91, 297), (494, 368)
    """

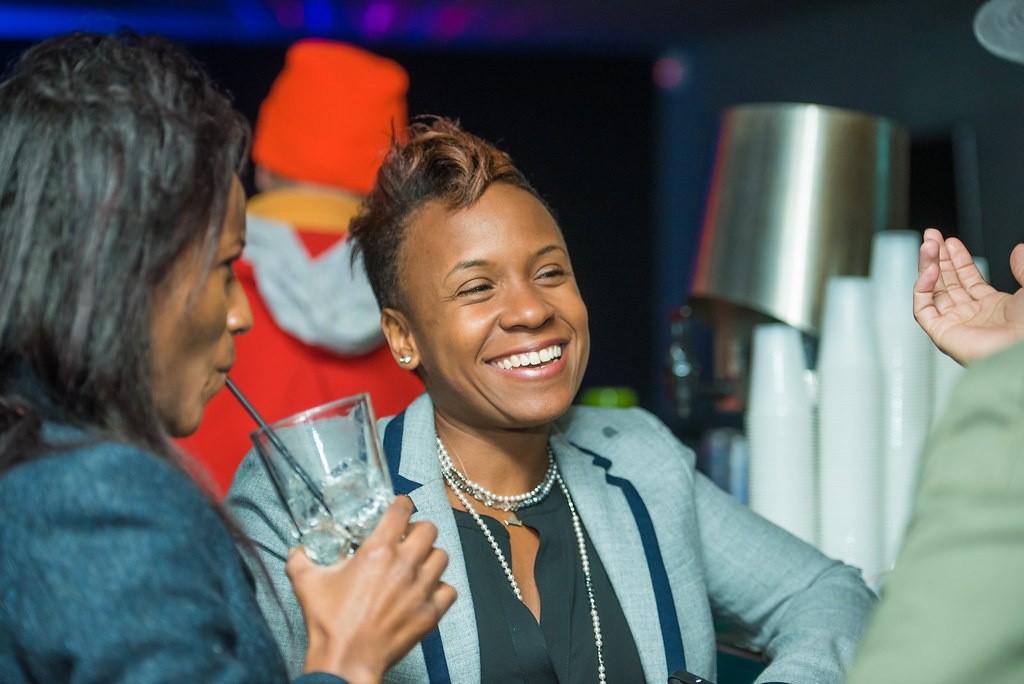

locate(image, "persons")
(221, 113), (878, 684)
(0, 33), (459, 684)
(849, 0), (1023, 683)
(169, 39), (426, 500)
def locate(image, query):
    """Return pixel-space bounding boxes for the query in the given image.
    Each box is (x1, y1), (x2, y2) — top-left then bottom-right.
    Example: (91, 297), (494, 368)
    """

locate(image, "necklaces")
(436, 436), (606, 684)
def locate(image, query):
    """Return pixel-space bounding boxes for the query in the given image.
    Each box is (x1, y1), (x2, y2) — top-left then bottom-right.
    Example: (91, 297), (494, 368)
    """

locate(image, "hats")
(249, 38), (413, 193)
(974, 0), (1024, 66)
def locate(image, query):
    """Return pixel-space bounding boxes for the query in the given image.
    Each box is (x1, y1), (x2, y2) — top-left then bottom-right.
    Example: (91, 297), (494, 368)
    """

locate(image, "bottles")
(668, 307), (698, 417)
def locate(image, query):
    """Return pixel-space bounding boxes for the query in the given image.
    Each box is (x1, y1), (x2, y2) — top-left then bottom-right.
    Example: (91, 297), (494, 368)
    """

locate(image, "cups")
(249, 391), (408, 568)
(744, 229), (989, 599)
